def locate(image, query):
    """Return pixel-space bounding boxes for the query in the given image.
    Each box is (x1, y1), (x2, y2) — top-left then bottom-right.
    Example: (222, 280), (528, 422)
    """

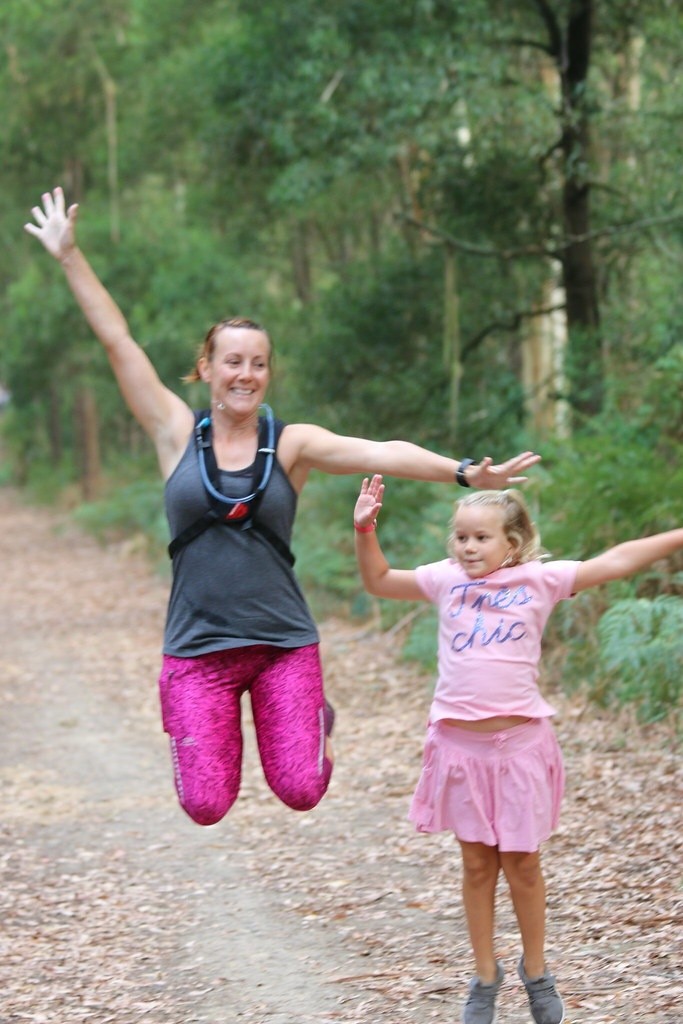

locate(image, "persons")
(355, 470), (683, 1024)
(25, 186), (542, 826)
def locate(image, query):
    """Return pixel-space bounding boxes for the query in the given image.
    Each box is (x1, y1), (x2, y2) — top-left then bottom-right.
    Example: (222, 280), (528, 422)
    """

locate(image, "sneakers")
(324, 699), (336, 735)
(463, 963), (505, 1024)
(517, 954), (565, 1024)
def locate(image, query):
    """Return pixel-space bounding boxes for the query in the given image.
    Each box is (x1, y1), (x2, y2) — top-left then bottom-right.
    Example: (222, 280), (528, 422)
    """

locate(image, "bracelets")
(456, 457), (477, 489)
(354, 519), (377, 534)
